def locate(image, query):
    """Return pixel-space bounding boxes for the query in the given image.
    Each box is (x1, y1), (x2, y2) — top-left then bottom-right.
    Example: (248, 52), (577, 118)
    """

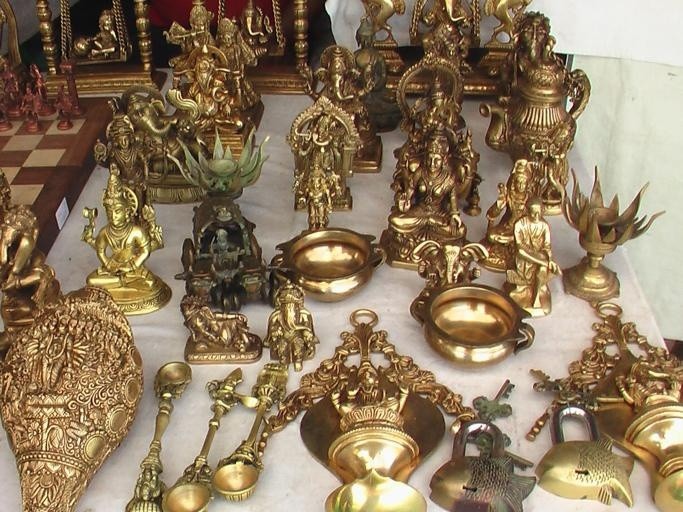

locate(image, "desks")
(0, 94), (683, 511)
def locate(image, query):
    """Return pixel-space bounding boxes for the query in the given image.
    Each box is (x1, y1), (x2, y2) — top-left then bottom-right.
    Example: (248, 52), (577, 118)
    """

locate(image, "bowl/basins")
(269, 227), (387, 304)
(410, 283), (534, 367)
